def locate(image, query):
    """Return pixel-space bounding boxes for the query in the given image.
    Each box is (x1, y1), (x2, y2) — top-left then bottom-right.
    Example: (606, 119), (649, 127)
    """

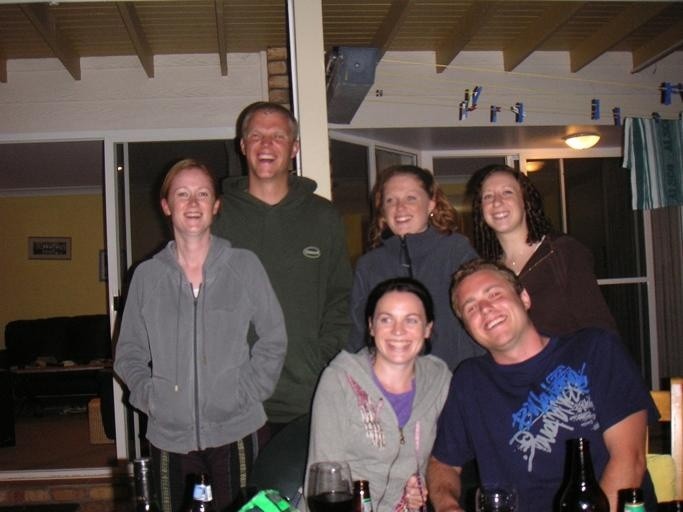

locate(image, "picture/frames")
(98, 249), (127, 282)
(28, 236), (72, 260)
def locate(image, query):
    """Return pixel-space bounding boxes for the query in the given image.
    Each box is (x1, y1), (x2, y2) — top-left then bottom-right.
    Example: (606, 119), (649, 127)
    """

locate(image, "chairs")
(644, 379), (682, 512)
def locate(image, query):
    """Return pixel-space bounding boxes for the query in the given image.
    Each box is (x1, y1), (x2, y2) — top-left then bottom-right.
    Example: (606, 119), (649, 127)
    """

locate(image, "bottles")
(133, 458), (158, 512)
(623, 487), (646, 512)
(191, 470), (216, 512)
(352, 480), (373, 512)
(555, 437), (610, 512)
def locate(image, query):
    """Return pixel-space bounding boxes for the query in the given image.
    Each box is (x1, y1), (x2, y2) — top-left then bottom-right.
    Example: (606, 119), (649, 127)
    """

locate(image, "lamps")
(561, 132), (601, 150)
(525, 160), (544, 172)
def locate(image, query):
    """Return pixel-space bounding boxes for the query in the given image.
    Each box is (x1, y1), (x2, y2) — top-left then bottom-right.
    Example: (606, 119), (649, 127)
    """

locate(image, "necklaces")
(499, 243), (534, 268)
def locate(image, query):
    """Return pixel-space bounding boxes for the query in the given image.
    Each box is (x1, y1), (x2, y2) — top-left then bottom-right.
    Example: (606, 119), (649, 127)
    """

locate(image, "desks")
(9, 362), (113, 444)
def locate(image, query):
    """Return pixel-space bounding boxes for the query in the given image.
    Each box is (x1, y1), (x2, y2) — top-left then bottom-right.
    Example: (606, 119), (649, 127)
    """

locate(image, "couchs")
(0, 314), (115, 448)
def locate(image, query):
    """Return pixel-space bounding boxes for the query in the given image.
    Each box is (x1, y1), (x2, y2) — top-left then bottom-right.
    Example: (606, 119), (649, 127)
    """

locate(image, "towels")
(620, 117), (681, 212)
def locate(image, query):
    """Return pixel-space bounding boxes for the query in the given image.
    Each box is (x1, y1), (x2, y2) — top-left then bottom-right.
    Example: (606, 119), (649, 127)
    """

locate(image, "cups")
(307, 463), (352, 511)
(475, 487), (518, 512)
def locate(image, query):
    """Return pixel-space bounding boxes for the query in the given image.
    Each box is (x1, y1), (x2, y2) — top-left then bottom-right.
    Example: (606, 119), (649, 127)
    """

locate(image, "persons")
(350, 165), (487, 372)
(425, 260), (647, 512)
(466, 164), (609, 337)
(299, 278), (451, 511)
(211, 101), (350, 508)
(113, 159), (286, 511)
